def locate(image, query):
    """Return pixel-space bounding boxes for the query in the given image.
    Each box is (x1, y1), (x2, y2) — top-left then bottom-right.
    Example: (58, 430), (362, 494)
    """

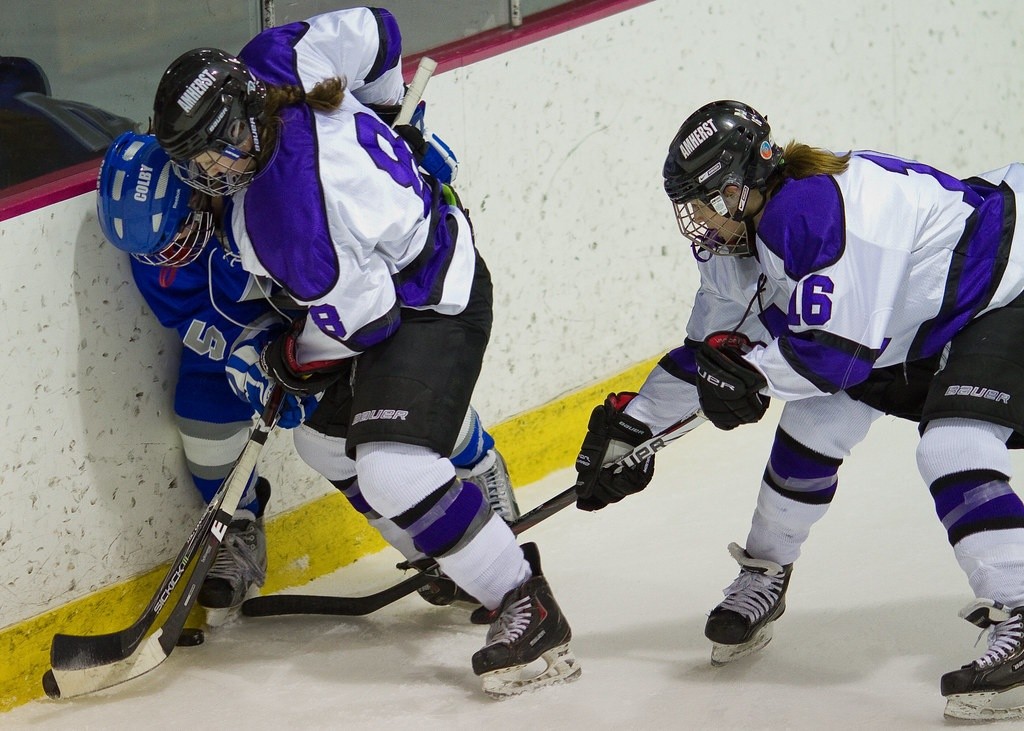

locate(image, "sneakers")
(469, 604), (499, 626)
(471, 541), (584, 695)
(704, 542), (793, 663)
(938, 597), (1024, 723)
(195, 475), (272, 626)
(455, 447), (519, 528)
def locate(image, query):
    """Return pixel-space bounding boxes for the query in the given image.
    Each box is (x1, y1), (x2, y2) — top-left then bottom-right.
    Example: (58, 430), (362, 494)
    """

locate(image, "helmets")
(150, 49), (281, 198)
(95, 133), (218, 268)
(662, 99), (784, 259)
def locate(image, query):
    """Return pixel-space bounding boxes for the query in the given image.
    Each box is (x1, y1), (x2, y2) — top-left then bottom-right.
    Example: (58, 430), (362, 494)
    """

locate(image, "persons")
(153, 6), (582, 701)
(575, 99), (1024, 727)
(96, 116), (521, 632)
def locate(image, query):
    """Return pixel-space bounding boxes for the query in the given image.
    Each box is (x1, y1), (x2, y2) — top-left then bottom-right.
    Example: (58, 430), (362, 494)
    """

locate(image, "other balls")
(175, 627), (205, 647)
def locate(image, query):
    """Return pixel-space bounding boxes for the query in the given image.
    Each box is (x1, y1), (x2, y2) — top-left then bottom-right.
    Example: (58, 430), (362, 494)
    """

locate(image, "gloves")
(574, 392), (658, 511)
(685, 330), (770, 432)
(257, 335), (338, 400)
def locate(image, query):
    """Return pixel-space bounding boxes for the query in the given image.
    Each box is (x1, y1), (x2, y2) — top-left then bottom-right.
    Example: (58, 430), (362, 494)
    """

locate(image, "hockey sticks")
(240, 403), (710, 616)
(47, 432), (251, 671)
(41, 54), (439, 700)
